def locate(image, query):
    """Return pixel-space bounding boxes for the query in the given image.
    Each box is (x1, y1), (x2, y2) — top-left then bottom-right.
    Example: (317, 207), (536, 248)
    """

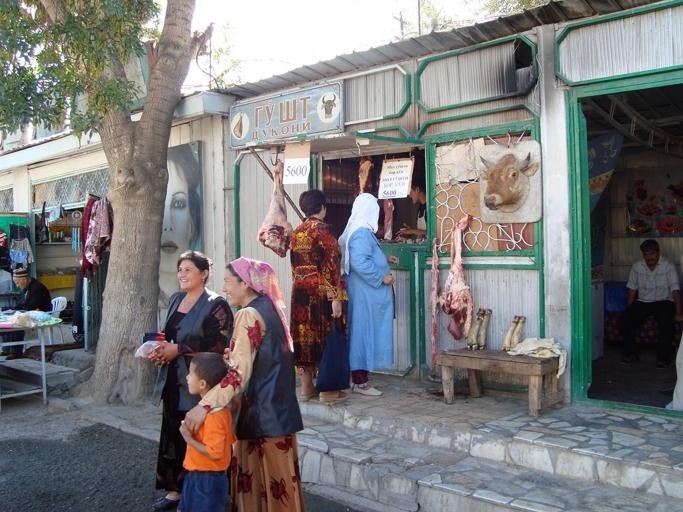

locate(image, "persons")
(394, 178), (427, 243)
(621, 239), (683, 369)
(337, 192), (399, 397)
(186, 256), (306, 512)
(146, 250), (235, 512)
(290, 188), (349, 403)
(5, 267), (52, 360)
(175, 353), (235, 512)
(157, 144), (201, 333)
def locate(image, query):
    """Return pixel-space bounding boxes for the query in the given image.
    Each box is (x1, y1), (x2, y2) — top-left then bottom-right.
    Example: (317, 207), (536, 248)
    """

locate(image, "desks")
(0, 315), (62, 410)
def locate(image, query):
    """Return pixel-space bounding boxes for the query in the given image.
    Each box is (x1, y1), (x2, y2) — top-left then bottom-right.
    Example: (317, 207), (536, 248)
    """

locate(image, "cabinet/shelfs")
(32, 211), (82, 321)
(590, 278), (605, 364)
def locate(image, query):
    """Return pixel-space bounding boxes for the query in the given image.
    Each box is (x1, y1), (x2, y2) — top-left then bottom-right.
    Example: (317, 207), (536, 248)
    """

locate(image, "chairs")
(38, 296), (66, 346)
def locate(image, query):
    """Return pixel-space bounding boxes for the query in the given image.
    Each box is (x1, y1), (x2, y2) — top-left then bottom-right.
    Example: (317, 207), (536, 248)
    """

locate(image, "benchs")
(429, 343), (565, 418)
(601, 279), (683, 346)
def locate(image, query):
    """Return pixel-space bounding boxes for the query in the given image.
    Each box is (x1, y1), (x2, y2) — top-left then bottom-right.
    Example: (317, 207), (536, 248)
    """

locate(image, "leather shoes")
(152, 495), (182, 511)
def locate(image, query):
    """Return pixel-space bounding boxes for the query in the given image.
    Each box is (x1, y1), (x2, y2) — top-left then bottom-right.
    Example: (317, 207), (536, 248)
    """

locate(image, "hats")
(11, 268), (30, 277)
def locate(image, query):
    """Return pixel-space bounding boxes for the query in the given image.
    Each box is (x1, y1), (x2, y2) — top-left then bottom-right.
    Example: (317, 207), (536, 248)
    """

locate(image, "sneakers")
(301, 384), (384, 404)
(620, 354), (670, 372)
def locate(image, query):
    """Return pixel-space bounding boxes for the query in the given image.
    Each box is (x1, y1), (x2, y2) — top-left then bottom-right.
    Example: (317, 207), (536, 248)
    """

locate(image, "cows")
(318, 93), (337, 118)
(477, 151), (541, 215)
